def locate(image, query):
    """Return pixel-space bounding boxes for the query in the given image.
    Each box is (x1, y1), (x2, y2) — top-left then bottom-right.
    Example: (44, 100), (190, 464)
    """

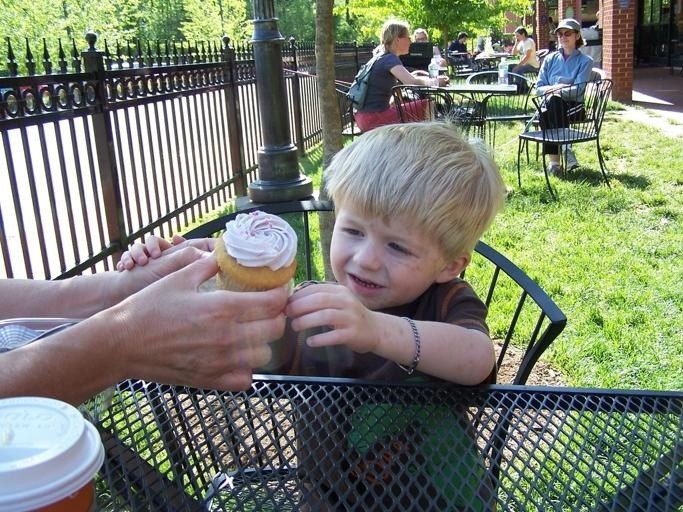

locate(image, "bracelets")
(395, 317), (422, 375)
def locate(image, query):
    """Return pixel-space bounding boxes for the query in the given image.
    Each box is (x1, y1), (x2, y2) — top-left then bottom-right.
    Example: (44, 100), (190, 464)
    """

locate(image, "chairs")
(465, 71), (535, 164)
(391, 85), (454, 123)
(520, 48), (549, 90)
(141, 199), (567, 512)
(517, 79), (613, 201)
(447, 89), (484, 139)
(335, 83), (362, 142)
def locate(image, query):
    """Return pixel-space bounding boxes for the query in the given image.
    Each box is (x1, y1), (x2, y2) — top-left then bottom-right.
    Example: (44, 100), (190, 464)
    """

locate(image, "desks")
(431, 84), (517, 96)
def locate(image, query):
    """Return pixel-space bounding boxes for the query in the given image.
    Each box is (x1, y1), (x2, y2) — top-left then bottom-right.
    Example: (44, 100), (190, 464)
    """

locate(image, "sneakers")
(564, 149), (579, 171)
(547, 161), (560, 174)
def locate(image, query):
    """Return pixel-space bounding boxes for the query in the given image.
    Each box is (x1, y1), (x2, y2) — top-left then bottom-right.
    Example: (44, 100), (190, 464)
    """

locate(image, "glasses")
(400, 36), (410, 41)
(556, 31), (576, 37)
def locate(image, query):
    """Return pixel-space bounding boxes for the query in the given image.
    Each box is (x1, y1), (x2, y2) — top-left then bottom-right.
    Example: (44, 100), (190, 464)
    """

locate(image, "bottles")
(497, 56), (508, 87)
(428, 57), (440, 89)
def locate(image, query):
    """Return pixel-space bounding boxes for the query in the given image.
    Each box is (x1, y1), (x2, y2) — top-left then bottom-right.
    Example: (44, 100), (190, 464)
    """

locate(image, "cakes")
(214, 209), (299, 293)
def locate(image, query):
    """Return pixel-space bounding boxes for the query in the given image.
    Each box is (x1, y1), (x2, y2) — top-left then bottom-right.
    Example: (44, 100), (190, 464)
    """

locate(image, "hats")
(554, 19), (580, 34)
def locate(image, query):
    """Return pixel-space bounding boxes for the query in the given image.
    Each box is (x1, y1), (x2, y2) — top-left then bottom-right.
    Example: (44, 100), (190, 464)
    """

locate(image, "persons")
(1, 238), (288, 408)
(352, 24), (450, 132)
(535, 19), (594, 175)
(116, 122), (504, 511)
(414, 28), (447, 75)
(549, 17), (556, 51)
(507, 28), (539, 95)
(448, 31), (472, 71)
(590, 10), (603, 45)
